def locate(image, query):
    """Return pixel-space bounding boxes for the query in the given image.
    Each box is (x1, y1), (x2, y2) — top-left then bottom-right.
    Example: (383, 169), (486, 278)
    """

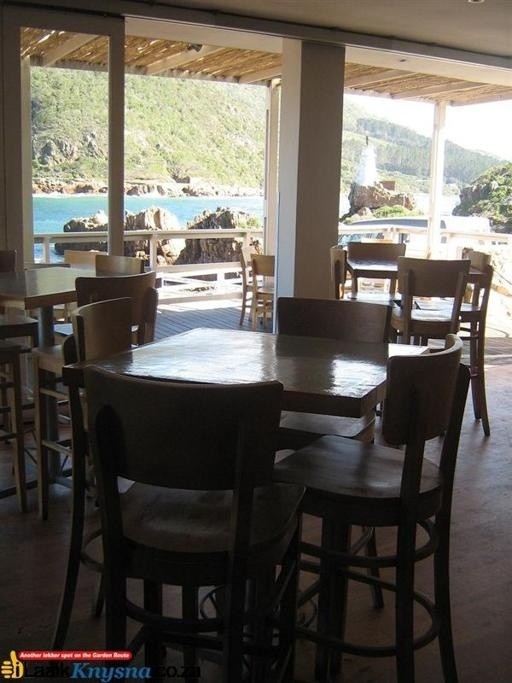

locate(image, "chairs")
(72, 296), (136, 363)
(348, 242), (405, 297)
(416, 246), (491, 420)
(238, 252), (264, 325)
(251, 254), (275, 331)
(94, 255), (144, 274)
(62, 249), (106, 268)
(33, 268), (160, 520)
(0, 250), (40, 348)
(459, 267), (494, 428)
(84, 363), (308, 678)
(331, 244), (348, 298)
(0, 343), (25, 515)
(276, 296), (390, 580)
(272, 332), (463, 682)
(390, 256), (472, 341)
